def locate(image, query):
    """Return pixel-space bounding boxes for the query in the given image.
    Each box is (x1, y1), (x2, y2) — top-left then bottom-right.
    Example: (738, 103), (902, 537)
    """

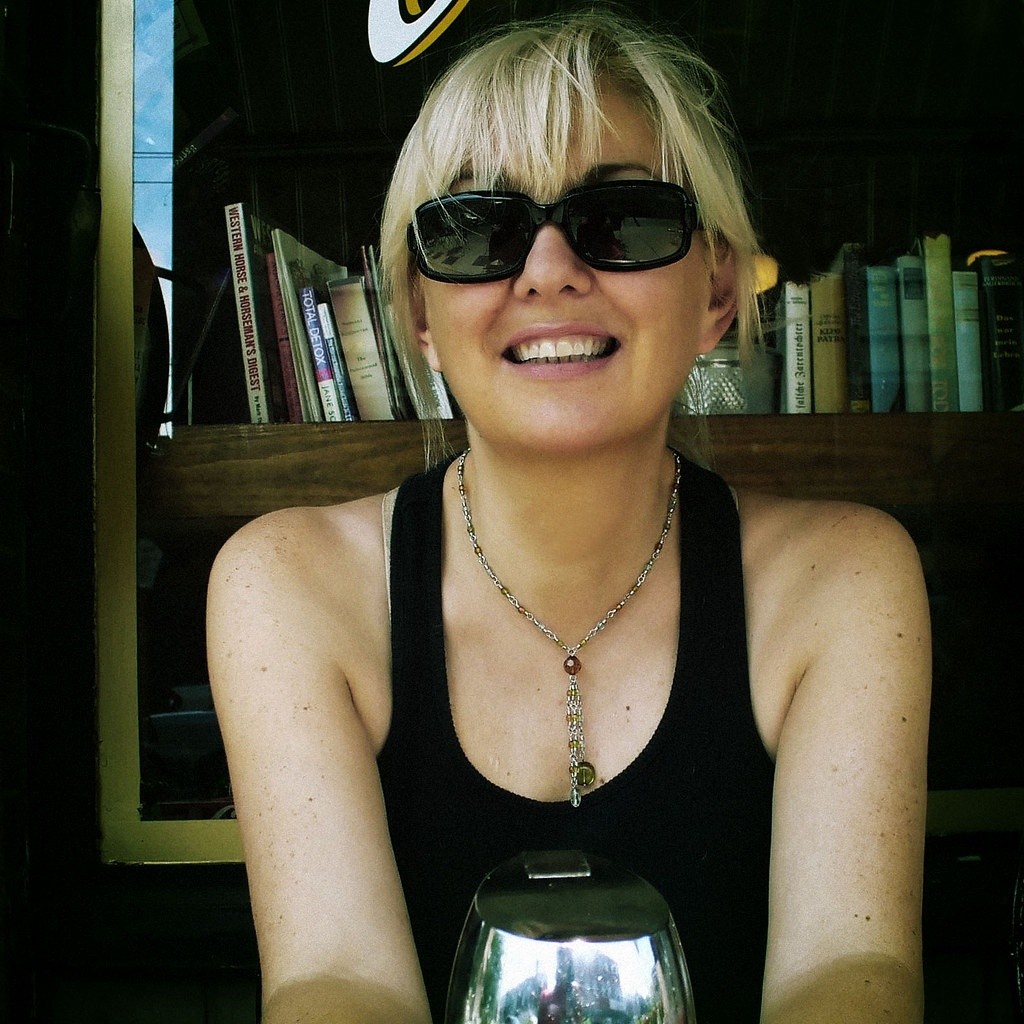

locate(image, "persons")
(207, 11), (931, 1024)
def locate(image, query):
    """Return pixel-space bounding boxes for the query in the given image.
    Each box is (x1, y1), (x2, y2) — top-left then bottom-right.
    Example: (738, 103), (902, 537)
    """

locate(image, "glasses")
(405, 180), (707, 284)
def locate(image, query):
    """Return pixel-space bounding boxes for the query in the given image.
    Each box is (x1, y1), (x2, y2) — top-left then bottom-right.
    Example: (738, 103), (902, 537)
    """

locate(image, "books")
(776, 232), (1024, 415)
(223, 203), (455, 423)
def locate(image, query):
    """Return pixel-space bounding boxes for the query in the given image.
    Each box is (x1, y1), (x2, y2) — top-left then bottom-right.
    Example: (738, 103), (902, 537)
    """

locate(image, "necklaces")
(455, 453), (681, 806)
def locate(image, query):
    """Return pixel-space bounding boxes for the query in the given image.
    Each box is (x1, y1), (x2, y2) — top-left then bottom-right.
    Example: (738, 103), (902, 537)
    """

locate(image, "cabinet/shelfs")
(96, 0), (1024, 866)
